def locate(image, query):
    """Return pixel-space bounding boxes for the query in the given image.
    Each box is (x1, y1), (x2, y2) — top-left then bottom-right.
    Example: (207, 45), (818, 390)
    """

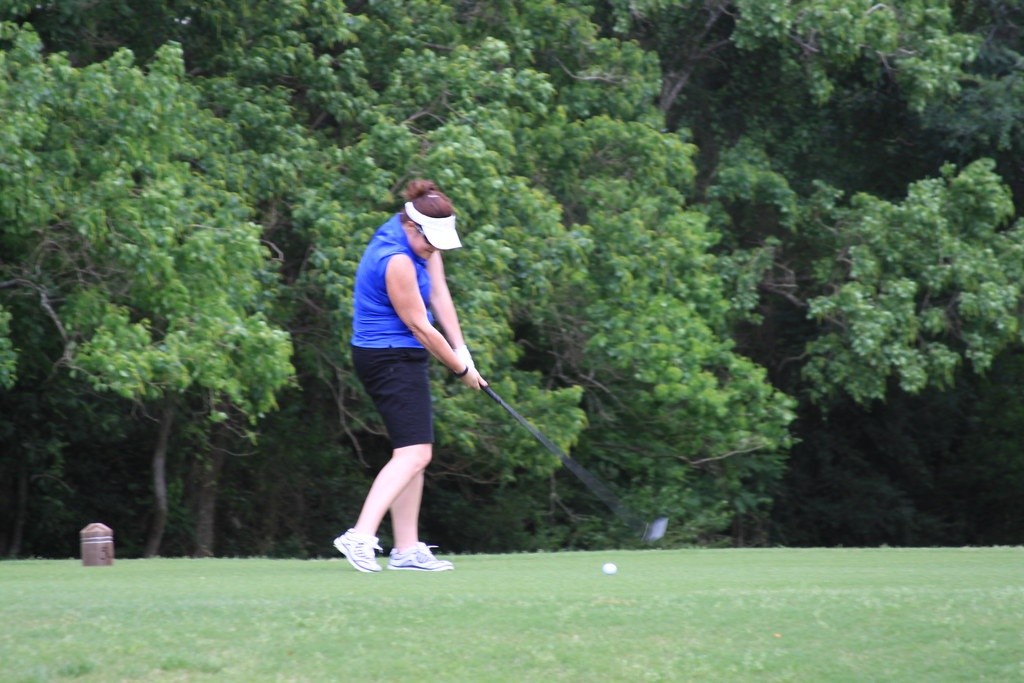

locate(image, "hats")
(406, 202), (462, 249)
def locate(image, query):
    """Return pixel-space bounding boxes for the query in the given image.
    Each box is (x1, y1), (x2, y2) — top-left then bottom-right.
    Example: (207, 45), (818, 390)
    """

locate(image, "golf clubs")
(480, 382), (670, 543)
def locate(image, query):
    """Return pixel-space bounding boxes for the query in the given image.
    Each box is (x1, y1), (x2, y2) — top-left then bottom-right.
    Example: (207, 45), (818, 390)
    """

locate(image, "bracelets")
(454, 365), (468, 378)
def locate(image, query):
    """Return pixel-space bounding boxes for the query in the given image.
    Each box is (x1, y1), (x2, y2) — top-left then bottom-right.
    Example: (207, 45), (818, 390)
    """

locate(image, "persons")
(333, 178), (489, 572)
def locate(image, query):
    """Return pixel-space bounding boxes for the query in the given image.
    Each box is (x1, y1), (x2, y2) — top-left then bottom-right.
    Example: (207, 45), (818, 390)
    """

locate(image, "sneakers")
(334, 528), (386, 572)
(388, 543), (454, 573)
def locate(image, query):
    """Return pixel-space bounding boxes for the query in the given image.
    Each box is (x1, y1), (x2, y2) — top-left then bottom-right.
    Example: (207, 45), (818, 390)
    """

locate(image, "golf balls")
(603, 562), (617, 576)
